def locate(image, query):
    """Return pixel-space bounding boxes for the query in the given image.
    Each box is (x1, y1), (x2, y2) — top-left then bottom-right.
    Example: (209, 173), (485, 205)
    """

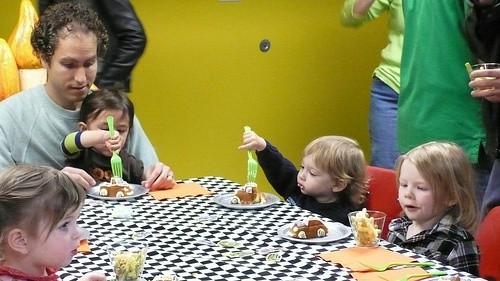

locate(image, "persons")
(60, 88), (144, 185)
(37, 0), (147, 92)
(0, 2), (176, 191)
(387, 141), (480, 277)
(341, 0), (500, 221)
(0, 165), (106, 281)
(238, 130), (370, 227)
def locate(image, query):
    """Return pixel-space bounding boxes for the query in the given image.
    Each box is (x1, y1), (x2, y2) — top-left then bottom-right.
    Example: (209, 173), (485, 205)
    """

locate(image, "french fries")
(351, 208), (382, 247)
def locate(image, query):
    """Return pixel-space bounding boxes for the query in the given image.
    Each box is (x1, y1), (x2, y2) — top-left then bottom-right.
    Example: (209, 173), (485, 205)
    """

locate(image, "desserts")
(99, 176), (134, 197)
(288, 216), (328, 238)
(230, 183), (266, 204)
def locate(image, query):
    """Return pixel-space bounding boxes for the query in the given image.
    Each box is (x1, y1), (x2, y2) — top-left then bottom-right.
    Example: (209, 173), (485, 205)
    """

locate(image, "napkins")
(77, 240), (92, 253)
(149, 183), (213, 201)
(319, 245), (417, 272)
(347, 265), (428, 281)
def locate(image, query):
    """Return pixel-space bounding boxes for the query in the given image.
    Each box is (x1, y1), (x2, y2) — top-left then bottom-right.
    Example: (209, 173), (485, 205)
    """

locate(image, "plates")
(277, 222), (352, 245)
(86, 183), (150, 201)
(213, 192), (279, 210)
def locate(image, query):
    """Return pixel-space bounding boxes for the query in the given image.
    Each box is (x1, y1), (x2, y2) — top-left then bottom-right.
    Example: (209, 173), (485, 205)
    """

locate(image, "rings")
(167, 175), (174, 178)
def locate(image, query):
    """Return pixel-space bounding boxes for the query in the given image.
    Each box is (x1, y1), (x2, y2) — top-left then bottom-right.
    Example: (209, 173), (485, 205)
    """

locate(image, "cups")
(348, 210), (387, 248)
(468, 64), (500, 90)
(107, 239), (149, 281)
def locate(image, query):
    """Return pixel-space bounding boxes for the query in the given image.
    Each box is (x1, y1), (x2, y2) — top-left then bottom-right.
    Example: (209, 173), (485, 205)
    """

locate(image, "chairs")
(366, 167), (401, 239)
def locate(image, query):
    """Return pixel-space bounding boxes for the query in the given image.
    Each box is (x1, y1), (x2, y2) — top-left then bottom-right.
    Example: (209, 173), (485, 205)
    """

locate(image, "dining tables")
(57, 176), (487, 281)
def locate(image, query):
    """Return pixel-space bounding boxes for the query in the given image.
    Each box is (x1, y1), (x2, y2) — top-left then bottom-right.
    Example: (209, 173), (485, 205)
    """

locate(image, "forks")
(105, 115), (123, 179)
(244, 126), (258, 183)
